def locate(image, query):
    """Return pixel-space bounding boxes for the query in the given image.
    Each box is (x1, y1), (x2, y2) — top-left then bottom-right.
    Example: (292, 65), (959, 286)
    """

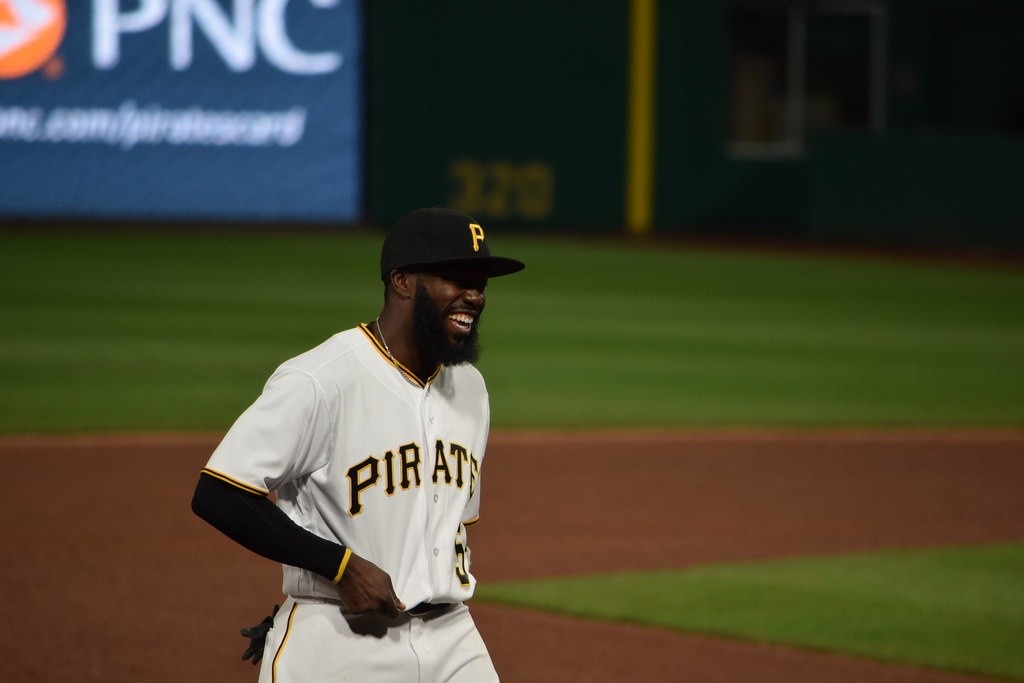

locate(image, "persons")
(183, 203), (520, 681)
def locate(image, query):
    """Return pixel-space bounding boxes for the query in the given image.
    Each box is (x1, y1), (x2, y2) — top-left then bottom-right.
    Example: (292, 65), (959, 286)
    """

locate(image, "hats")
(380, 205), (526, 281)
